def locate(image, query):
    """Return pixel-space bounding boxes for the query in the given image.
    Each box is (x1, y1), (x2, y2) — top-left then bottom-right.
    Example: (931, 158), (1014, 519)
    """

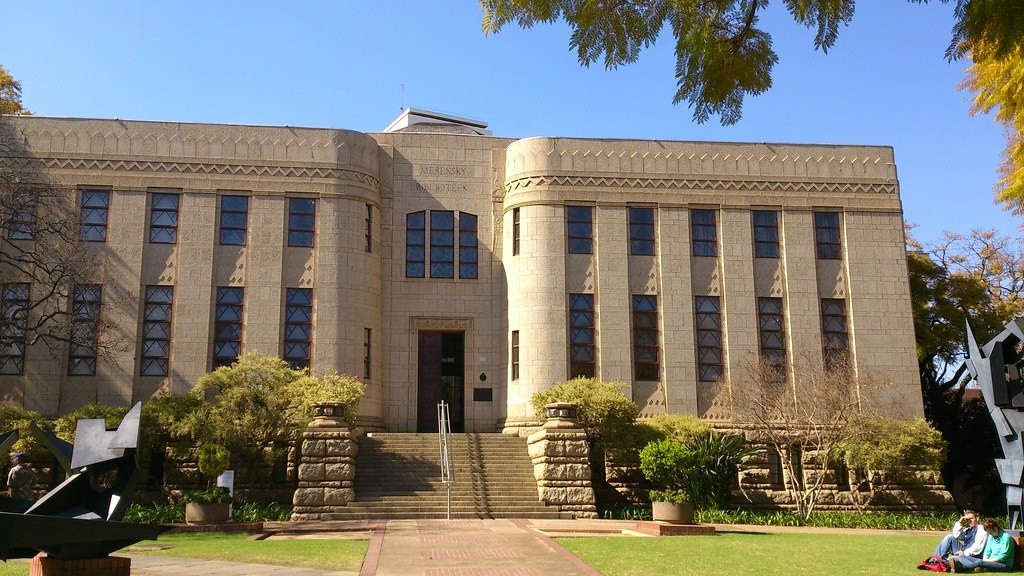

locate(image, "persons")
(933, 509), (988, 572)
(963, 516), (1017, 574)
(8, 452), (35, 501)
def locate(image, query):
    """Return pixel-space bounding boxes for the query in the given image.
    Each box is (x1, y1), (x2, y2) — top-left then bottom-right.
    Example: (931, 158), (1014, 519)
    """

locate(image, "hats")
(11, 453), (26, 459)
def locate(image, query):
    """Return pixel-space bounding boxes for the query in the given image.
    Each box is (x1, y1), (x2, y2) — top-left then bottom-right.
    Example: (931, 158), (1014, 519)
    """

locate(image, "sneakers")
(974, 567), (989, 573)
(948, 557), (959, 573)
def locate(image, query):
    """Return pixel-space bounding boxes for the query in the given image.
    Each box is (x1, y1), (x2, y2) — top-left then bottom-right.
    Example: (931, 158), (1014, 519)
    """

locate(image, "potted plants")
(639, 435), (700, 525)
(183, 444), (234, 524)
